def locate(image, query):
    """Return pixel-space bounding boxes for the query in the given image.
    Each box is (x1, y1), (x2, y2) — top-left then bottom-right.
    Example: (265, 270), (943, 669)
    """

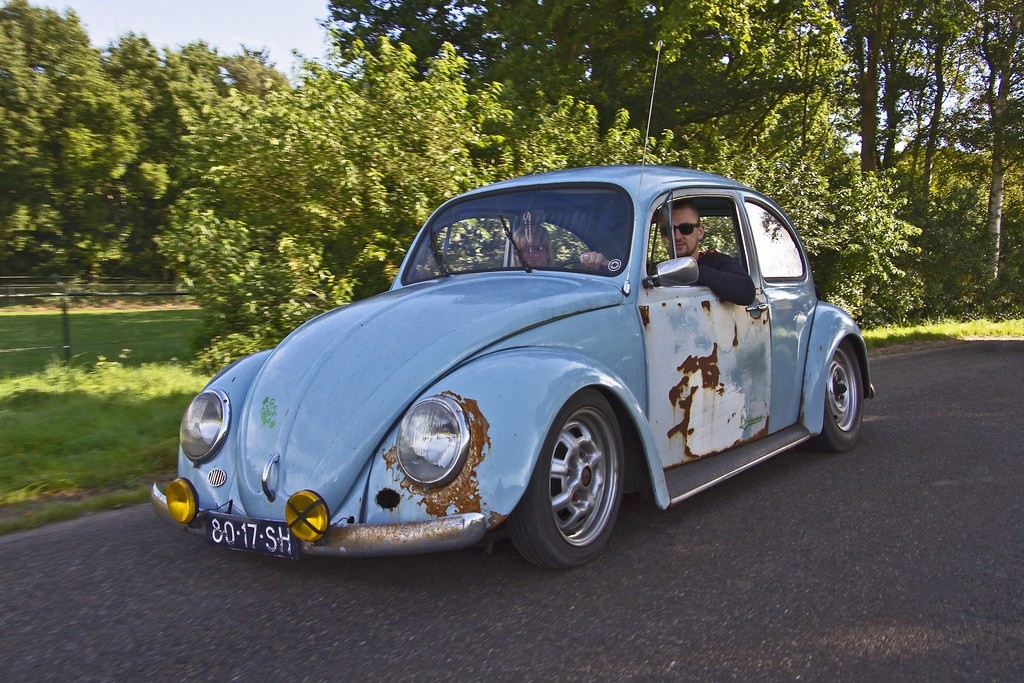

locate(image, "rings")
(583, 255), (590, 258)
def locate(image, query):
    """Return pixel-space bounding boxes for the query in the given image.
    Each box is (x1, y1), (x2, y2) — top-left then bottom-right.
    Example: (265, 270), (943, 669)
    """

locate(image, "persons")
(511, 223), (553, 268)
(580, 198), (756, 306)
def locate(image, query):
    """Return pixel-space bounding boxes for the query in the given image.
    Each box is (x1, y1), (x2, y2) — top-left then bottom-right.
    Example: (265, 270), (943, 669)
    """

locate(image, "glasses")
(660, 223), (699, 237)
(513, 244), (548, 256)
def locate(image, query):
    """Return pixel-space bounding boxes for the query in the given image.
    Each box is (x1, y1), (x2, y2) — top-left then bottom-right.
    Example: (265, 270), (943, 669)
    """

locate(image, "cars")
(149, 164), (874, 574)
